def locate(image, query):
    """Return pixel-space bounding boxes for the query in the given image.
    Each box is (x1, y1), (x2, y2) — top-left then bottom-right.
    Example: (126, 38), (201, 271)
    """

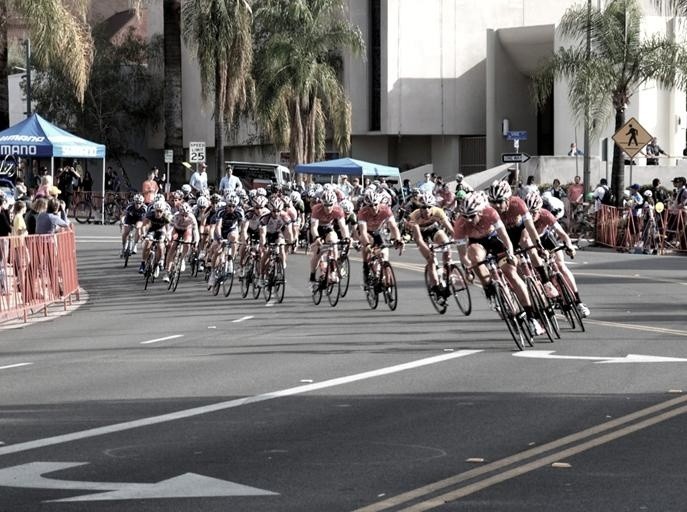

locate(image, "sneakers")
(384, 292), (394, 301)
(309, 257), (347, 286)
(431, 274), (451, 298)
(120, 243), (286, 286)
(489, 281), (591, 335)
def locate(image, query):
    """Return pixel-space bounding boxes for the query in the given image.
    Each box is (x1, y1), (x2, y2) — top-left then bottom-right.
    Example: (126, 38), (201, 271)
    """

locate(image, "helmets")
(412, 180), (565, 220)
(455, 173), (464, 181)
(133, 179), (389, 215)
(585, 192), (593, 202)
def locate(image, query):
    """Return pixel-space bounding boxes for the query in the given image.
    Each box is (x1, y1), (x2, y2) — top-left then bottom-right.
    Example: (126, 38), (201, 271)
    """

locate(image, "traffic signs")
(190, 142), (206, 163)
(502, 153), (531, 163)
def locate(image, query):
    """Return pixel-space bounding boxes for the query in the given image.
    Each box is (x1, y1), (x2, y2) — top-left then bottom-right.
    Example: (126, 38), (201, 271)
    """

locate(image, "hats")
(629, 184), (653, 197)
(199, 163), (207, 168)
(49, 186), (62, 195)
(671, 178), (680, 182)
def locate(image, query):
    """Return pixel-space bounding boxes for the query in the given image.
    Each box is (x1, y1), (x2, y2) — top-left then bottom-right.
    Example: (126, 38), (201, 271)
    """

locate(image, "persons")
(624, 177), (686, 254)
(568, 142), (583, 156)
(399, 172), (474, 305)
(120, 168), (200, 281)
(593, 178), (611, 218)
(454, 175), (591, 334)
(0, 155), (94, 295)
(623, 152), (636, 165)
(646, 138), (670, 165)
(189, 163), (250, 286)
(106, 168), (131, 211)
(241, 181), (405, 301)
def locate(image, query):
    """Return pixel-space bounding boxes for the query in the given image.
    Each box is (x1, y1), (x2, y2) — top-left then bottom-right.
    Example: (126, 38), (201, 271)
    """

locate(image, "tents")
(293, 157), (405, 203)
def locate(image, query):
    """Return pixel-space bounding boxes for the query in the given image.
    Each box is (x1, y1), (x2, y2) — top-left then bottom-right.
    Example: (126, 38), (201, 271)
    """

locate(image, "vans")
(224, 161), (291, 193)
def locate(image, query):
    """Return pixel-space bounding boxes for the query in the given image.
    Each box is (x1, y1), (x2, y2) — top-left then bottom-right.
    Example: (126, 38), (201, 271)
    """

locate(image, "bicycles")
(74, 191), (121, 225)
(310, 235), (350, 307)
(142, 235), (298, 304)
(119, 222), (143, 269)
(326, 237), (361, 298)
(462, 241), (586, 352)
(424, 240), (472, 317)
(362, 240), (403, 312)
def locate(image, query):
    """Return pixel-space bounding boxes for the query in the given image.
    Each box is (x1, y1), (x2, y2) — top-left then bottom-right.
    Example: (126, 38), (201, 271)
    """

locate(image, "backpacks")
(597, 185), (614, 205)
